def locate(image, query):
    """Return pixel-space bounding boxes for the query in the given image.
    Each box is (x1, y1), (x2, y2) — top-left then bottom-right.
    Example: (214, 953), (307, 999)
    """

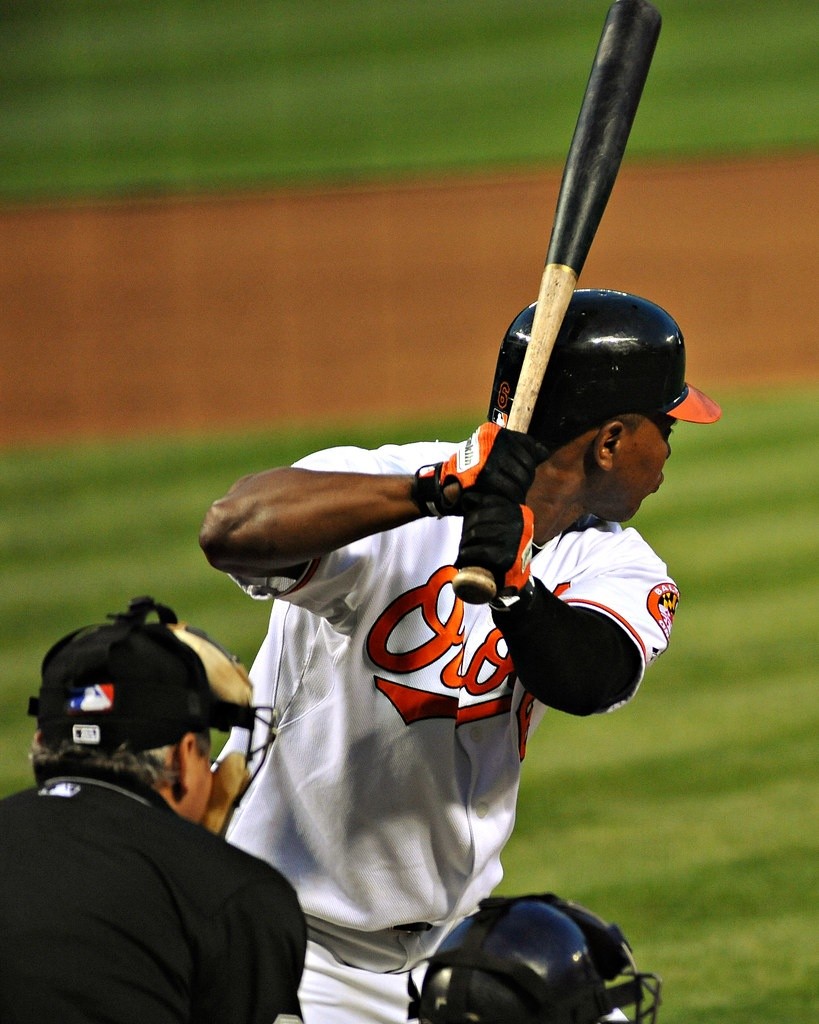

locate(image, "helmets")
(419, 894), (609, 1024)
(488, 288), (721, 441)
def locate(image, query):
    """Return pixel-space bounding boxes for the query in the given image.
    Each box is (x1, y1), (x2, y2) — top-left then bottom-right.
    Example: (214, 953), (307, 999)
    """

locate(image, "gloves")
(412, 422), (550, 521)
(456, 494), (535, 611)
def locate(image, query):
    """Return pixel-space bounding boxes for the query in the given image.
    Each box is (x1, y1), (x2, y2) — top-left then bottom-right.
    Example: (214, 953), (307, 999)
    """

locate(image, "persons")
(197, 292), (723, 1024)
(0, 595), (311, 1023)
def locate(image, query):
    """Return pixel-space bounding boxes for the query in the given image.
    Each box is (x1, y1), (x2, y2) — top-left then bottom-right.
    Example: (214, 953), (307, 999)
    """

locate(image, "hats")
(28, 620), (213, 745)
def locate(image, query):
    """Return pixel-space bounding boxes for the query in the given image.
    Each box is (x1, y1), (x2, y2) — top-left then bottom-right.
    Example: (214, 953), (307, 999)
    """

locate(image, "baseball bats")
(450, 0), (671, 608)
